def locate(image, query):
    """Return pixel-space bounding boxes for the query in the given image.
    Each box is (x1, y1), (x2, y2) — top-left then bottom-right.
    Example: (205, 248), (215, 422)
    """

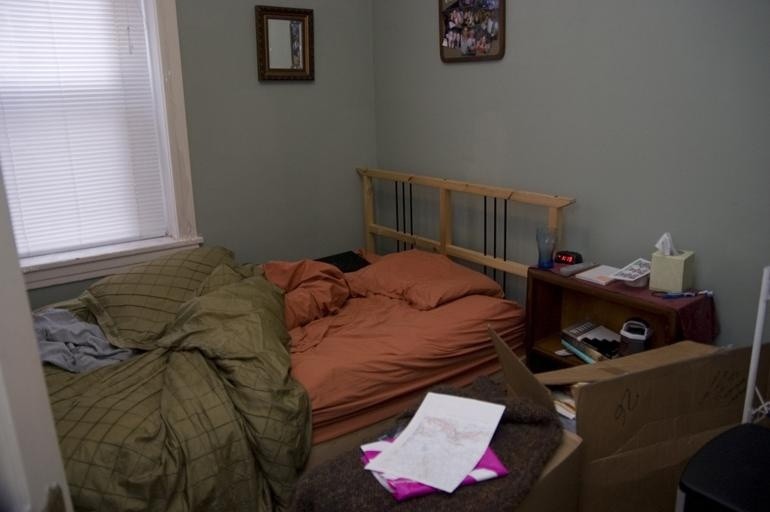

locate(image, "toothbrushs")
(663, 290), (714, 298)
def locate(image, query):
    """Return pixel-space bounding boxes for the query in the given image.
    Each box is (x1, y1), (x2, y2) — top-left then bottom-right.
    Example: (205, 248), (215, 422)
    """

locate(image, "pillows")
(344, 250), (504, 310)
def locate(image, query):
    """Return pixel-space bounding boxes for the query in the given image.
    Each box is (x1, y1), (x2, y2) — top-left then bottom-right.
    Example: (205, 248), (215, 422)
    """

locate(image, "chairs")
(676, 265), (770, 512)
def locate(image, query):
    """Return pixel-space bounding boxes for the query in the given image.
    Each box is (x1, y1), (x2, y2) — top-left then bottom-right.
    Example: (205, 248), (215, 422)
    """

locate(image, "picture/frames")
(289, 20), (303, 69)
(438, 0), (506, 62)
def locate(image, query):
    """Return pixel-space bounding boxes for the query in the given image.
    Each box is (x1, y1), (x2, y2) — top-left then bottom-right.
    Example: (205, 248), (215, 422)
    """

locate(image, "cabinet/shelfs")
(524, 260), (712, 379)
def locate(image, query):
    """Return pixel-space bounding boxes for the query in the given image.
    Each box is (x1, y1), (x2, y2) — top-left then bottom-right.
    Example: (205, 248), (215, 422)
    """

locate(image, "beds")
(26, 166), (575, 512)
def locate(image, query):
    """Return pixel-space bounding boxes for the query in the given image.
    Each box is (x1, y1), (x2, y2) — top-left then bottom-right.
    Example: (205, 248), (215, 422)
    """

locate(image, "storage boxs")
(485, 324), (770, 512)
(514, 429), (583, 512)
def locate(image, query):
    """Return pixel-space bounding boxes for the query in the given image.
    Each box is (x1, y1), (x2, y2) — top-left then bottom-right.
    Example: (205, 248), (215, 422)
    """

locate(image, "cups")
(536, 227), (559, 269)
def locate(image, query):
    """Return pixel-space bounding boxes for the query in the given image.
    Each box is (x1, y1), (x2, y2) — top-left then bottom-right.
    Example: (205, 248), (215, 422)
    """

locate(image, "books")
(558, 318), (622, 366)
(575, 264), (622, 286)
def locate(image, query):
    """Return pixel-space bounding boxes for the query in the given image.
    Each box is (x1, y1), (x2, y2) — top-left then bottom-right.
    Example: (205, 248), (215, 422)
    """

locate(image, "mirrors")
(255, 5), (315, 81)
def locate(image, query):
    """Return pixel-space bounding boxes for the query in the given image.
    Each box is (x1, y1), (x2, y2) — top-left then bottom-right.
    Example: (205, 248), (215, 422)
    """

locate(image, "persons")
(445, 6), (493, 57)
